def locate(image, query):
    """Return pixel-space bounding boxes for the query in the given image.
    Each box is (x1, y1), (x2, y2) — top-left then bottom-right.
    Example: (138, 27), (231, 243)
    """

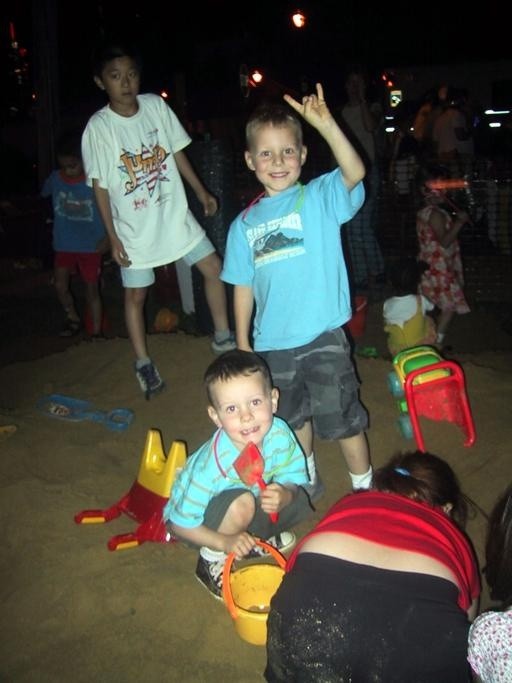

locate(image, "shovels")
(233, 440), (278, 523)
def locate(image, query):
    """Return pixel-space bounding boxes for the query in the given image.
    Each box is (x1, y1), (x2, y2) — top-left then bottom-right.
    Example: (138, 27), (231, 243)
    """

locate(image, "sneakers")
(242, 528), (296, 560)
(133, 358), (163, 394)
(193, 552), (238, 603)
(210, 334), (254, 356)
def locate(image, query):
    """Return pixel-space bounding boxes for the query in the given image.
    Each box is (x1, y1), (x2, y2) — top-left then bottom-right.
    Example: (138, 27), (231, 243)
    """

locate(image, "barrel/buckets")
(223, 540), (287, 647)
(343, 294), (369, 337)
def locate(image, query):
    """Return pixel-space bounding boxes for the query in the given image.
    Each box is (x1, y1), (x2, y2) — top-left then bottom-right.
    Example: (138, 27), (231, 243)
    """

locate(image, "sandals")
(57, 311), (83, 338)
